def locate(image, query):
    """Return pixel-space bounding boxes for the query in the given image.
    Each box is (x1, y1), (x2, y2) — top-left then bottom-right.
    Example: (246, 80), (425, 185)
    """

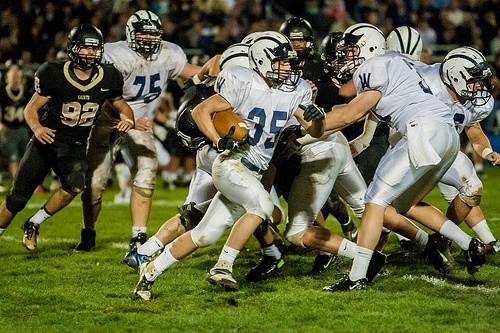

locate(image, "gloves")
(217, 126), (249, 151)
(298, 104), (325, 122)
(279, 123), (306, 159)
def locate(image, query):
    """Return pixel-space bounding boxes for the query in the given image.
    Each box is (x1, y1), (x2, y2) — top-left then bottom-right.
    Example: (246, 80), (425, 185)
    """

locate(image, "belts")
(241, 157), (265, 175)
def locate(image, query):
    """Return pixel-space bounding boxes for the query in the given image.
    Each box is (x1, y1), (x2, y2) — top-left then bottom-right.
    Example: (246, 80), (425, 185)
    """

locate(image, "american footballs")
(211, 108), (249, 142)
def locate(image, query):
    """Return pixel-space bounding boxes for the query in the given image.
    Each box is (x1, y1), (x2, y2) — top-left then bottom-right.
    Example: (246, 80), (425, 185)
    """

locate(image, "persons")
(123, 16), (500, 303)
(73, 10), (203, 253)
(0, 66), (28, 196)
(0, 24), (135, 251)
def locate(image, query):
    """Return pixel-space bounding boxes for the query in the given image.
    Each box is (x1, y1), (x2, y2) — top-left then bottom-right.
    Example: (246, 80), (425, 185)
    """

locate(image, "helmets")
(125, 10), (162, 54)
(67, 24), (104, 69)
(439, 46), (492, 100)
(218, 17), (422, 82)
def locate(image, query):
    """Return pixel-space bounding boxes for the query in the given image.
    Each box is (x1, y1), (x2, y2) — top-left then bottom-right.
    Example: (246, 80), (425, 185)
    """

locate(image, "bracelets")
(481, 148), (493, 159)
(124, 119), (134, 125)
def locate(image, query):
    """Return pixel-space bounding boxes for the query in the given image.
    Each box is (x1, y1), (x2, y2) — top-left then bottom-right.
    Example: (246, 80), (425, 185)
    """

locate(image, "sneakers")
(177, 201), (205, 232)
(305, 253), (342, 277)
(366, 249), (387, 282)
(130, 232), (147, 252)
(21, 215), (40, 250)
(246, 253), (285, 281)
(388, 233), (500, 273)
(124, 247), (151, 274)
(205, 260), (238, 291)
(323, 273), (368, 291)
(341, 216), (358, 243)
(134, 262), (158, 302)
(74, 228), (96, 253)
